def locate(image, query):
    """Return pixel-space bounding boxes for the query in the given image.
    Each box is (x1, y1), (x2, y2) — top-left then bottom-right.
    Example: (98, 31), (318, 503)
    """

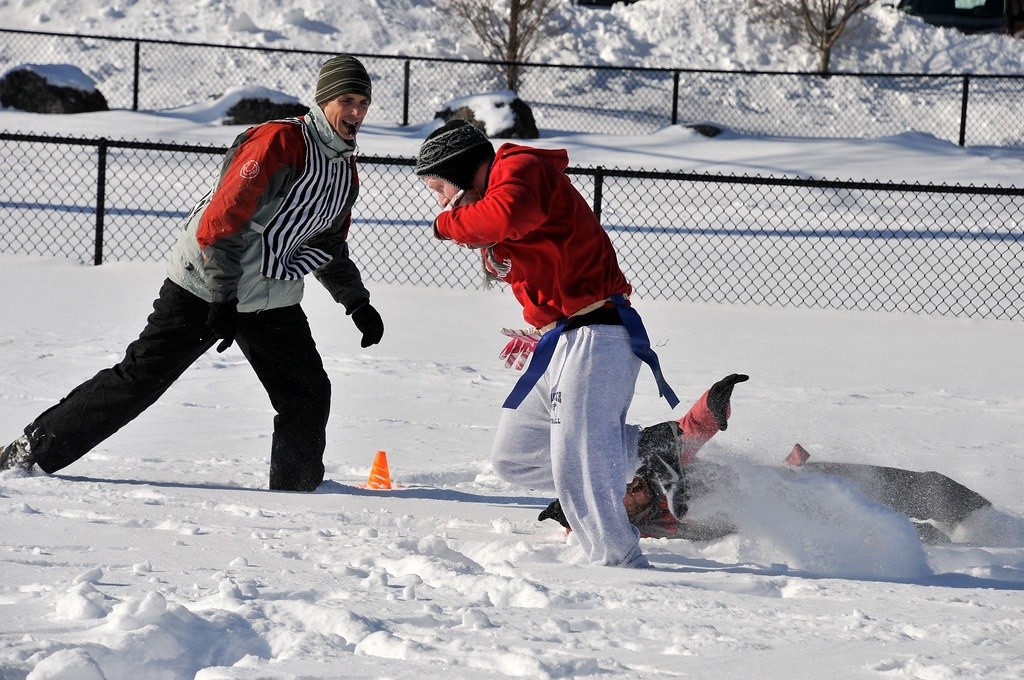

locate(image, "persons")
(0, 54), (384, 492)
(417, 118), (681, 568)
(538, 371), (995, 546)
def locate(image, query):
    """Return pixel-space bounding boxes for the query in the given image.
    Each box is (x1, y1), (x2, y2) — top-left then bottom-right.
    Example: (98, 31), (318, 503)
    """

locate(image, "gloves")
(498, 326), (543, 370)
(537, 499), (571, 529)
(206, 297), (239, 352)
(433, 211), (454, 240)
(707, 373), (749, 431)
(345, 297), (384, 348)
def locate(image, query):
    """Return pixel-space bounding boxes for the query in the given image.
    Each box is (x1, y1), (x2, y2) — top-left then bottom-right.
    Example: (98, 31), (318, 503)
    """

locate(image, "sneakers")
(640, 422), (690, 522)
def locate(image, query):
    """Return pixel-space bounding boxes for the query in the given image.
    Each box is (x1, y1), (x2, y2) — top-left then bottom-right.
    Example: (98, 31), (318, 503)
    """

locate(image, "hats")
(314, 54), (372, 105)
(415, 119), (496, 192)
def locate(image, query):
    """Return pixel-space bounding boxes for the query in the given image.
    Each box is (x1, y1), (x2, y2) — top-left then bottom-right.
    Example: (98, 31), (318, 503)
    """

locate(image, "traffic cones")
(367, 451), (391, 489)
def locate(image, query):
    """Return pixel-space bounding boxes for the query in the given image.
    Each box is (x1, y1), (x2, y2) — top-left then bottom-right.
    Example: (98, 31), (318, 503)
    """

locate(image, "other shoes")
(0, 436), (36, 477)
